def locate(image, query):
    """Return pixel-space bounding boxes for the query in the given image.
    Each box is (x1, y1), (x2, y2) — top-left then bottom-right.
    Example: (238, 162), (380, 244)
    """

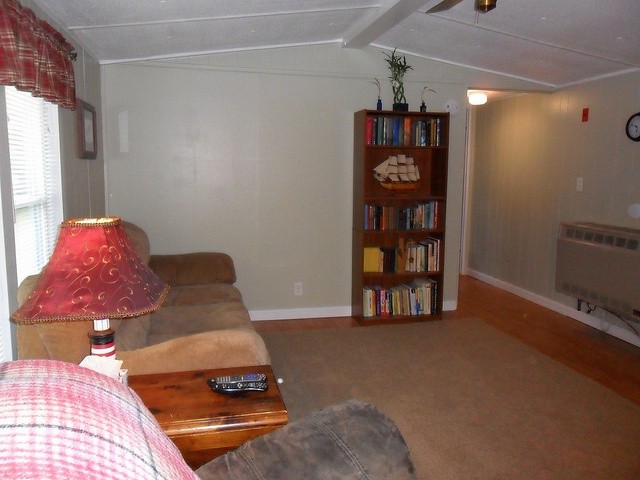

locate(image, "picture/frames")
(76, 100), (99, 158)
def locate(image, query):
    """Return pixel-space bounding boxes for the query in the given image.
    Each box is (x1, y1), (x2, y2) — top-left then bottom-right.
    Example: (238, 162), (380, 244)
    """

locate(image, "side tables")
(128, 366), (288, 465)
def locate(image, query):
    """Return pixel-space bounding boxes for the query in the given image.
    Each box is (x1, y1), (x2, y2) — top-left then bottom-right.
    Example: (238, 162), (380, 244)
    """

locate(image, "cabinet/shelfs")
(352, 109), (451, 325)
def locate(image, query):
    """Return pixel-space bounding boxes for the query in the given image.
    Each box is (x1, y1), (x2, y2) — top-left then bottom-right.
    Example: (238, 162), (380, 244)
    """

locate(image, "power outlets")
(577, 177), (583, 191)
(581, 108), (590, 122)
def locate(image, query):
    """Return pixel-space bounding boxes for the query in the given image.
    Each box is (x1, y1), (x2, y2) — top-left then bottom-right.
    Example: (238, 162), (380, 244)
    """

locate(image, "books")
(364, 236), (440, 272)
(363, 200), (438, 231)
(364, 277), (438, 317)
(365, 115), (442, 148)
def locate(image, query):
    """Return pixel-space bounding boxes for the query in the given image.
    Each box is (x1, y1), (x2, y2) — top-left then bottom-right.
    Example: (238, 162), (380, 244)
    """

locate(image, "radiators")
(553, 224), (640, 321)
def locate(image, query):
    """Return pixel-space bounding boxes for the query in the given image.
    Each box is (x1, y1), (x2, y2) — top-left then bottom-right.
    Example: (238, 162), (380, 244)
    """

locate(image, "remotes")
(207, 373), (268, 385)
(213, 381), (268, 395)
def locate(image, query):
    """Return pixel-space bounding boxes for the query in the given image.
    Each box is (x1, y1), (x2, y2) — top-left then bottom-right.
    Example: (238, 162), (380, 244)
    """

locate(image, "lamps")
(469, 93), (487, 106)
(10, 218), (170, 361)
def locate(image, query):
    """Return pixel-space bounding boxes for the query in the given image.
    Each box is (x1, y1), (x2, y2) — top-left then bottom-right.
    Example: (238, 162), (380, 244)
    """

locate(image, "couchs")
(0, 361), (414, 479)
(16, 214), (270, 376)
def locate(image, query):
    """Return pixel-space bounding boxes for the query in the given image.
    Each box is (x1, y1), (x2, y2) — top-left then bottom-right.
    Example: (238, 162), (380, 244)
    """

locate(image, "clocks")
(625, 113), (640, 141)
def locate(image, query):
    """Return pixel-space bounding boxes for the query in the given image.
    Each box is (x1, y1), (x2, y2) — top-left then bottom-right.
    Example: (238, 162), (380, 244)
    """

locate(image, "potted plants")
(384, 48), (413, 112)
(372, 78), (384, 111)
(420, 86), (436, 112)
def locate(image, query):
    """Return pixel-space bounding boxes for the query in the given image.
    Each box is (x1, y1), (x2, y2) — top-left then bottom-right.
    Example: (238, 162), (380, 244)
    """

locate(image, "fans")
(424, 0), (495, 15)
